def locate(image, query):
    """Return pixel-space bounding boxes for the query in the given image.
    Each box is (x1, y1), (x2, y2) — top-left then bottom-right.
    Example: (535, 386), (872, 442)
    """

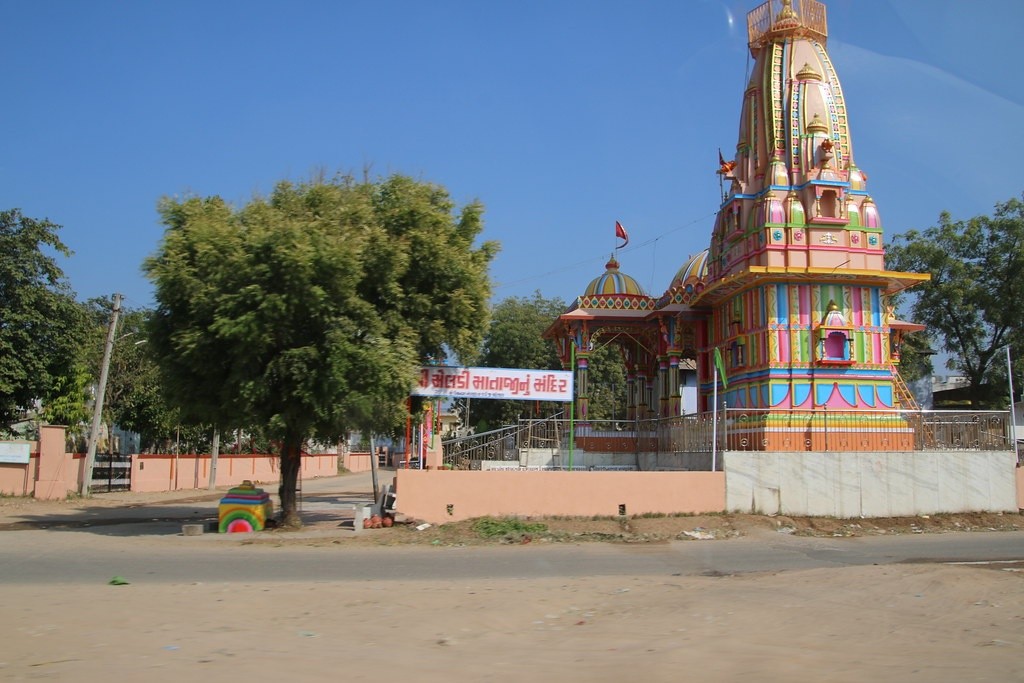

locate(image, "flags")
(714, 347), (728, 389)
(719, 150), (727, 165)
(616, 221), (628, 249)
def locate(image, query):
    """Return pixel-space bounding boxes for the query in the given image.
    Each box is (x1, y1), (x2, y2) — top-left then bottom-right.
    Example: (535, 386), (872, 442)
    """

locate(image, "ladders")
(517, 400), (564, 471)
(891, 363), (934, 444)
